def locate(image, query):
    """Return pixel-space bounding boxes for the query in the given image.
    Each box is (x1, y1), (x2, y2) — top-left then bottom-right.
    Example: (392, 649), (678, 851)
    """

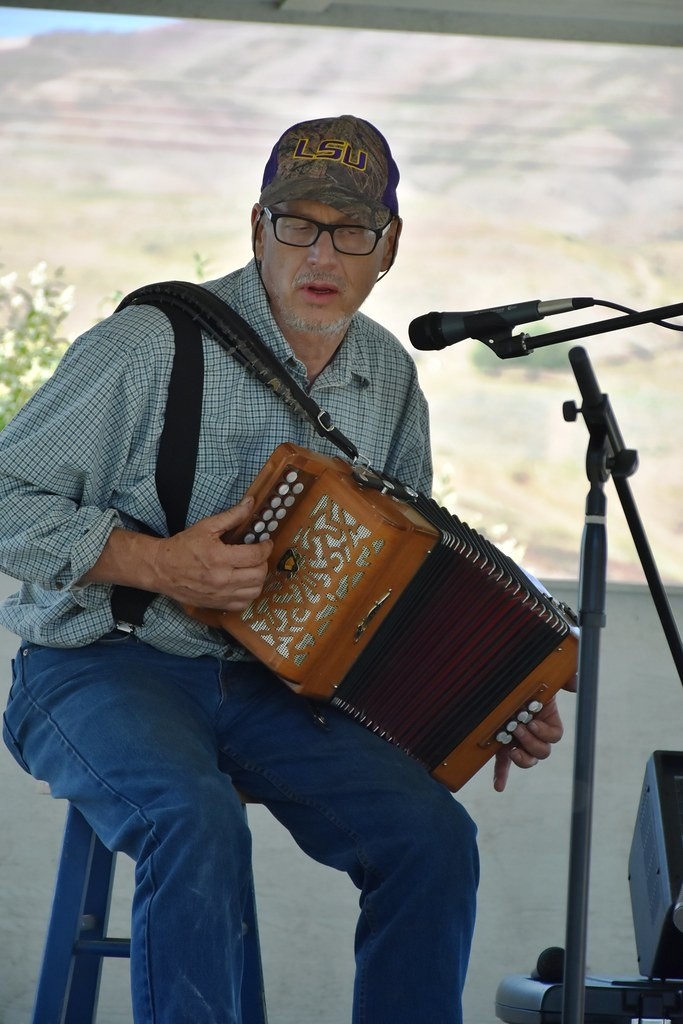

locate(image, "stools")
(32, 779), (269, 1024)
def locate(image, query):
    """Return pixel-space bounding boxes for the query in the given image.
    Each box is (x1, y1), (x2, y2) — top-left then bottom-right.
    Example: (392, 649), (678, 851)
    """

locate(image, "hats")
(259, 115), (400, 231)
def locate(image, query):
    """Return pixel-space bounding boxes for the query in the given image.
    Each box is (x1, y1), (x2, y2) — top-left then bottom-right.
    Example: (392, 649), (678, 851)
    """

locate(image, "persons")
(0, 114), (578, 1024)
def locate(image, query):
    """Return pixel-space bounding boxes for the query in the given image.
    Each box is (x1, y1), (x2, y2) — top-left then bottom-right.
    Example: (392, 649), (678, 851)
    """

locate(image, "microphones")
(408, 297), (594, 351)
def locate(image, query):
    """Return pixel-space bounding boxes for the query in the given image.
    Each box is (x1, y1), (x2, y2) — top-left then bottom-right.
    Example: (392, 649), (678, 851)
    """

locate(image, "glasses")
(260, 204), (395, 256)
